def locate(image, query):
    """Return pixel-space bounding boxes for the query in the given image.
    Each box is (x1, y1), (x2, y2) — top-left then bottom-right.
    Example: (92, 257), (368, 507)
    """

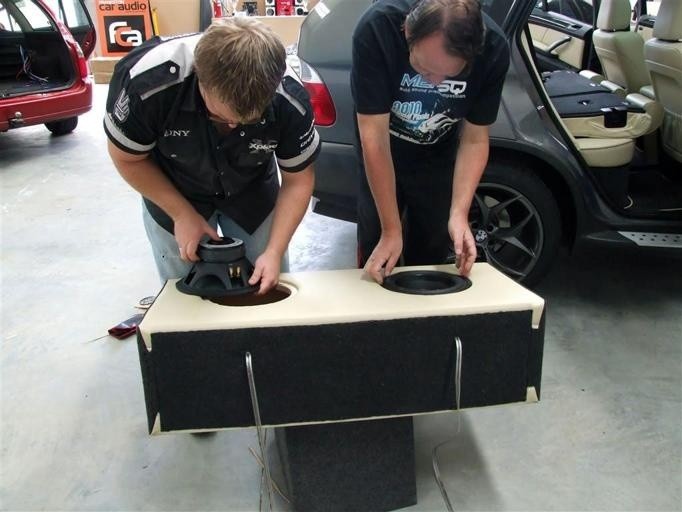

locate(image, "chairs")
(535, 0), (682, 167)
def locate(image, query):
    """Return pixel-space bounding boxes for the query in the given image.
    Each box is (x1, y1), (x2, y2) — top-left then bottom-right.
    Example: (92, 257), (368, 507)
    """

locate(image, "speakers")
(135, 238), (548, 434)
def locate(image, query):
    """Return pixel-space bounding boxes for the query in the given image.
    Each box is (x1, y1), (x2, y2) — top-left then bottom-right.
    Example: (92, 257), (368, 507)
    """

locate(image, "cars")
(0, 0), (97, 137)
(283, 0), (682, 294)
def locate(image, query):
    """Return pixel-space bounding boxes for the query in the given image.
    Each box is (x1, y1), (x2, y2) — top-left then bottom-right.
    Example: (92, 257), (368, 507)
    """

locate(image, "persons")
(345, 0), (512, 288)
(101, 12), (324, 440)
